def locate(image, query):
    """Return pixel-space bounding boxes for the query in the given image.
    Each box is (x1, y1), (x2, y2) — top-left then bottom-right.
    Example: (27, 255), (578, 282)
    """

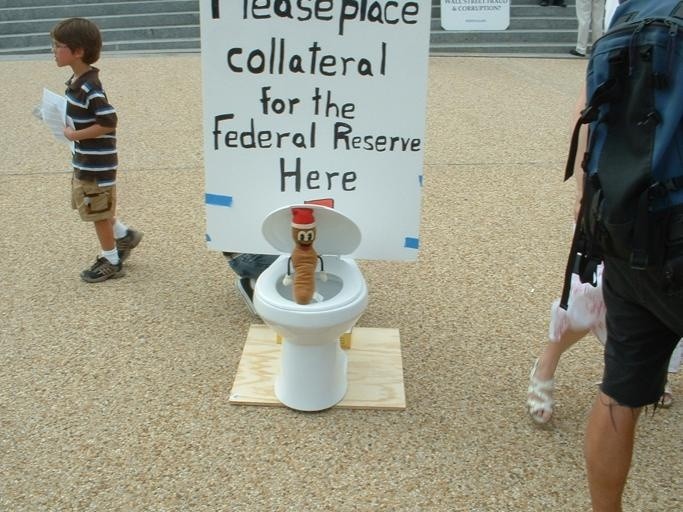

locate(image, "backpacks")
(563, 1), (683, 271)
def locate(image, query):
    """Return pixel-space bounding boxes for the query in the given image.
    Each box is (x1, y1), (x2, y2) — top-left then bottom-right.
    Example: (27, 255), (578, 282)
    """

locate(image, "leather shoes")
(570, 48), (584, 56)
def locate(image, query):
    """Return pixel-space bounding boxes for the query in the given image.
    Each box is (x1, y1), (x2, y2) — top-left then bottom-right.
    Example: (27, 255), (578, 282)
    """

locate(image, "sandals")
(527, 360), (553, 423)
(659, 382), (674, 405)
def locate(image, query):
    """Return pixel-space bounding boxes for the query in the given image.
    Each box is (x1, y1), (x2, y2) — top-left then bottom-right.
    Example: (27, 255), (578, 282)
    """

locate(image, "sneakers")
(235, 276), (258, 315)
(81, 257), (125, 283)
(113, 229), (143, 262)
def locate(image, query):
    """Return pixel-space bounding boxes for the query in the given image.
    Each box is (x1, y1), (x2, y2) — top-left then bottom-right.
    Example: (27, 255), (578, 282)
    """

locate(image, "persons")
(223, 252), (279, 318)
(50, 15), (145, 284)
(525, 1), (683, 511)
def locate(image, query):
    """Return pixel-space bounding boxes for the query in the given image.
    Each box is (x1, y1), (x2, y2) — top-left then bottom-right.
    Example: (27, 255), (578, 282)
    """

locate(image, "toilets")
(250, 203), (369, 412)
